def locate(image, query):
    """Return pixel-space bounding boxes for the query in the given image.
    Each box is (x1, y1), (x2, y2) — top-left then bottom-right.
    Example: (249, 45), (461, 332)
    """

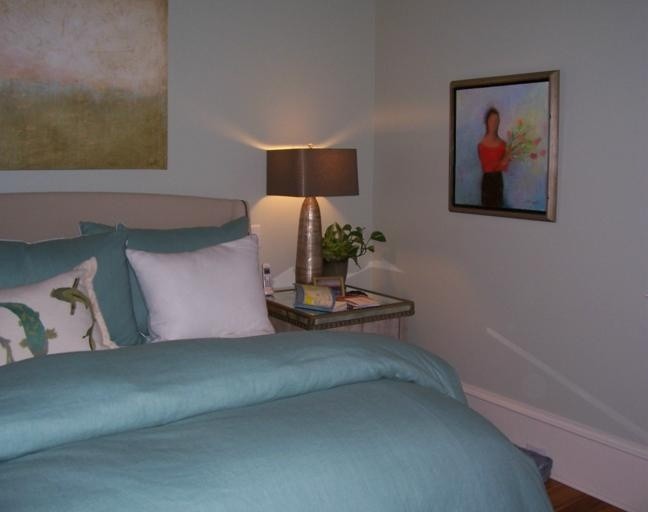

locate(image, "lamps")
(264, 145), (363, 284)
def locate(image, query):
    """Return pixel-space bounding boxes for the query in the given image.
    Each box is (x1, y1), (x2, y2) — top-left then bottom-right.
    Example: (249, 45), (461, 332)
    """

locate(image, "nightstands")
(259, 281), (417, 342)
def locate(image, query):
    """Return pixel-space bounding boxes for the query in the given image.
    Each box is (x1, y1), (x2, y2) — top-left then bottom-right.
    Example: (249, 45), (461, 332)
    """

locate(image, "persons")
(477, 108), (511, 209)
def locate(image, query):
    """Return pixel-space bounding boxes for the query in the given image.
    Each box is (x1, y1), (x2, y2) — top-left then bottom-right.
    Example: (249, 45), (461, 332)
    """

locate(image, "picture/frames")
(447, 69), (564, 224)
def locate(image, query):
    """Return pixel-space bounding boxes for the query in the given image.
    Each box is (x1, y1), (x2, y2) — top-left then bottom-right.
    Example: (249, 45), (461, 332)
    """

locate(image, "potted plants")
(321, 220), (385, 285)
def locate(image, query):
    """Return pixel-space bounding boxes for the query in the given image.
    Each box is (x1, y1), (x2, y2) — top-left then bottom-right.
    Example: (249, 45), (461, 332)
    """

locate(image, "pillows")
(1, 222), (142, 352)
(79, 215), (253, 347)
(1, 255), (124, 368)
(123, 231), (276, 342)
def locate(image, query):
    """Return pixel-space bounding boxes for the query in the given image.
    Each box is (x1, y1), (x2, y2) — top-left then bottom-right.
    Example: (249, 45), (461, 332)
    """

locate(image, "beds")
(1, 189), (560, 512)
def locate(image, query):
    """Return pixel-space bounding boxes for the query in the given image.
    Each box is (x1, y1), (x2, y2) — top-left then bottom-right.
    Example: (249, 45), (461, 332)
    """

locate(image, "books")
(337, 296), (380, 310)
(293, 281), (348, 313)
